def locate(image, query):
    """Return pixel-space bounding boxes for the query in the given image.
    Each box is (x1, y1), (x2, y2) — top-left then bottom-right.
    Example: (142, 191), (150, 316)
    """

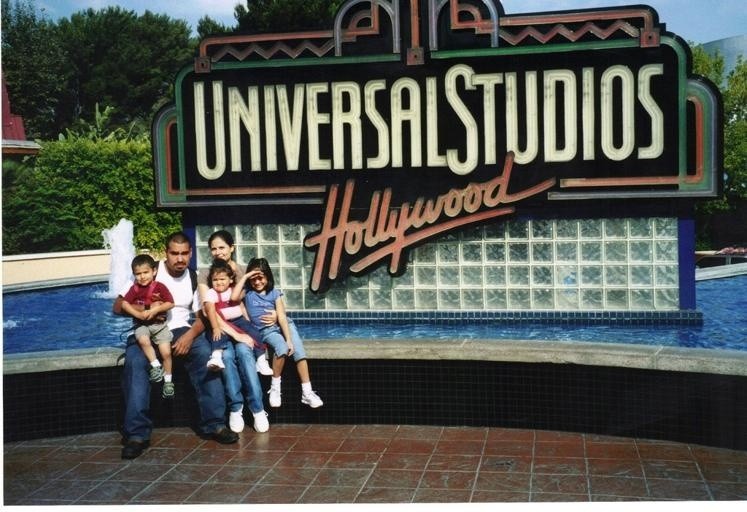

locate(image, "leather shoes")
(205, 425), (239, 444)
(121, 440), (151, 459)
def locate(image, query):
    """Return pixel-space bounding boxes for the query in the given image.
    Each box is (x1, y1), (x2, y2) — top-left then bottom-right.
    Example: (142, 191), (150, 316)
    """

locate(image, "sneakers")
(207, 358), (226, 372)
(268, 387), (282, 407)
(161, 381), (176, 400)
(255, 359), (274, 377)
(229, 403), (245, 433)
(149, 364), (166, 384)
(252, 409), (271, 432)
(301, 390), (324, 409)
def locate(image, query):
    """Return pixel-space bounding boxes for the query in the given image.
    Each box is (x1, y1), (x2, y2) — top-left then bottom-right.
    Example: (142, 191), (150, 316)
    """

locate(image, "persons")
(121, 254), (175, 400)
(197, 230), (279, 433)
(230, 257), (324, 409)
(112, 231), (240, 459)
(201, 258), (274, 376)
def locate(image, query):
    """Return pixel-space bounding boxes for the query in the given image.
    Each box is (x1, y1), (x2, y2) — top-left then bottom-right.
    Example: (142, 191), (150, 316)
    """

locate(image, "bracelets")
(145, 304), (150, 310)
